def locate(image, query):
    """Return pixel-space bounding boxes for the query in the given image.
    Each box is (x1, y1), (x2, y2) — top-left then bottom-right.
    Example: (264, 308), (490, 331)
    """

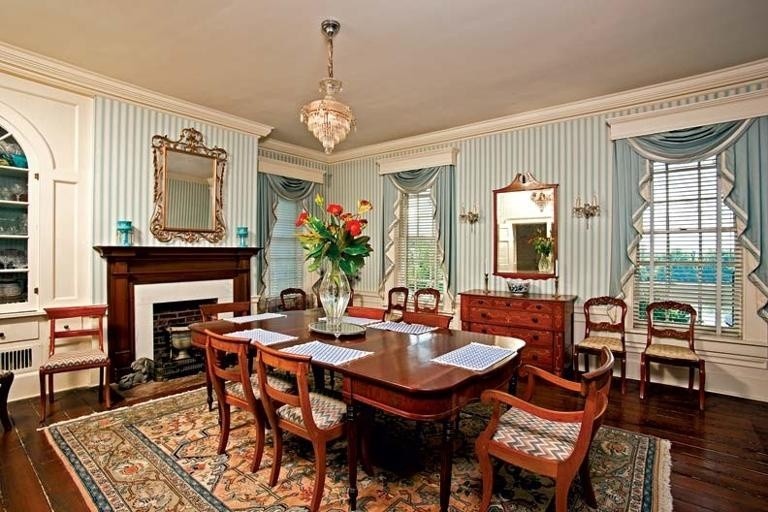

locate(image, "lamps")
(299, 19), (356, 155)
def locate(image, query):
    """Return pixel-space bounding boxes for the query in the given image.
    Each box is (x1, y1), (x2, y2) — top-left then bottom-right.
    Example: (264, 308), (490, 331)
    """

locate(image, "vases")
(537, 255), (552, 273)
(319, 258), (352, 326)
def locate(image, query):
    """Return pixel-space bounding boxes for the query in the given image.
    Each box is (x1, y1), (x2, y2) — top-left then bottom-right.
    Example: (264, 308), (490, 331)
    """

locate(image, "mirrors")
(492, 171), (559, 281)
(151, 127), (228, 243)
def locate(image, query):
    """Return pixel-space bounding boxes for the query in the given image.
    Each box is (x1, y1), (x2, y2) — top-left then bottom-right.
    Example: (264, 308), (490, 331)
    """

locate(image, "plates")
(308, 321), (367, 336)
(0, 280), (22, 298)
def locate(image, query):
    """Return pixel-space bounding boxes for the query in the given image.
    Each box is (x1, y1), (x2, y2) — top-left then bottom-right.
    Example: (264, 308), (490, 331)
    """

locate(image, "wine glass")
(0, 181), (25, 201)
(0, 249), (28, 269)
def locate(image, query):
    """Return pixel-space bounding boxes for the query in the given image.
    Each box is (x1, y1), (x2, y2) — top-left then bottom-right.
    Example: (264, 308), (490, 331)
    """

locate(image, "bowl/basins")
(506, 280), (530, 296)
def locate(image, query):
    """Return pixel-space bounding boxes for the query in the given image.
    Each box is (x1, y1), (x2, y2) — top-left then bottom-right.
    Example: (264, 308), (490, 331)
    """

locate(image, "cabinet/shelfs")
(0, 127), (38, 315)
(458, 288), (578, 378)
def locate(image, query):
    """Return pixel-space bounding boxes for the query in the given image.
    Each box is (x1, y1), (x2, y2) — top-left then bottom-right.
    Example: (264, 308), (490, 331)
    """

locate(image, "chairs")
(386, 288), (410, 313)
(415, 288), (441, 314)
(639, 299), (706, 413)
(204, 328), (297, 472)
(280, 288), (306, 308)
(254, 341), (376, 511)
(474, 345), (616, 511)
(574, 295), (628, 396)
(38, 304), (113, 425)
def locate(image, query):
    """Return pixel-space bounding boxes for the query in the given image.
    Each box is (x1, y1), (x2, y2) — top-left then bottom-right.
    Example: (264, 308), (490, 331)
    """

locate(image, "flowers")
(527, 236), (554, 268)
(293, 192), (377, 302)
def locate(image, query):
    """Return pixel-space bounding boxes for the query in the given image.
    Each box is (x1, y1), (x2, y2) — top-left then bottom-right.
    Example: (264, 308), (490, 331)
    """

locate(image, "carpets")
(44, 368), (675, 512)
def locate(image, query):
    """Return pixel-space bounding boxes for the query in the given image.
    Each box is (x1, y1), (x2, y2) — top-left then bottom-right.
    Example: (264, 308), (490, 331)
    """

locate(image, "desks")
(187, 306), (526, 512)
